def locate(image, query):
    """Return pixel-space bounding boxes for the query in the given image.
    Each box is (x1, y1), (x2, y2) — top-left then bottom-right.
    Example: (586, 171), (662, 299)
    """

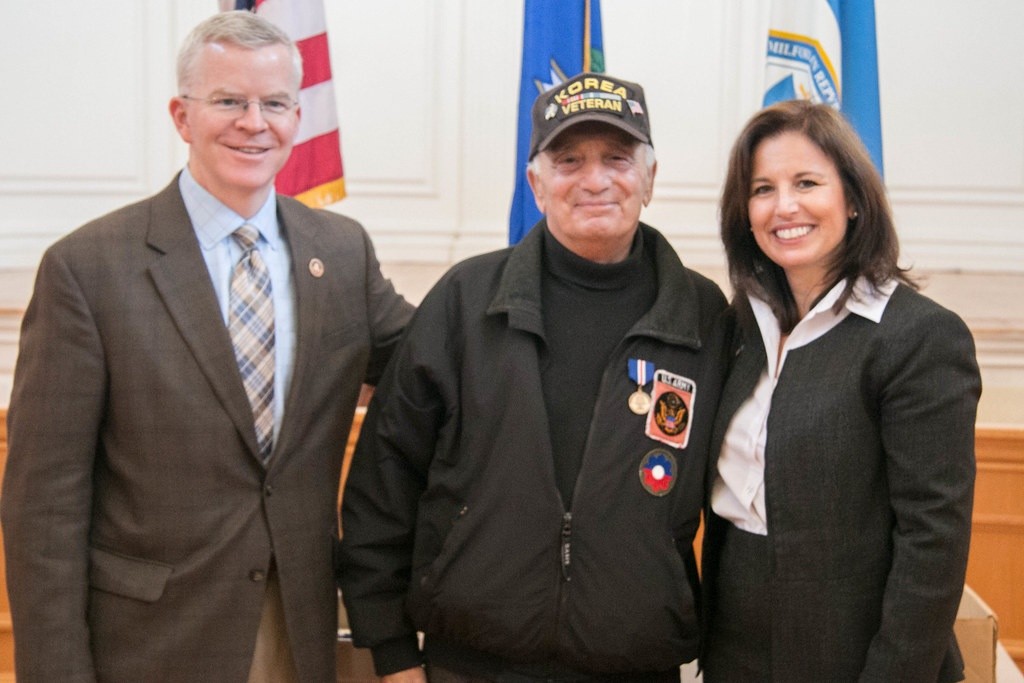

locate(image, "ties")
(229, 223), (275, 468)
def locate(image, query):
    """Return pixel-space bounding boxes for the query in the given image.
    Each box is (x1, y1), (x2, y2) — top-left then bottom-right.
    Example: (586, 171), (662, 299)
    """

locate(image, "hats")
(528, 72), (654, 167)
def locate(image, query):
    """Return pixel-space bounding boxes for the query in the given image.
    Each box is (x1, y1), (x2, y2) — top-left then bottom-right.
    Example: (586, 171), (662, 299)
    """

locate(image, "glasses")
(182, 96), (298, 114)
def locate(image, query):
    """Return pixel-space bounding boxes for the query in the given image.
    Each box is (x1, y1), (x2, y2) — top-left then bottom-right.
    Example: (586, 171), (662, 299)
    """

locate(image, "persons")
(0, 10), (420, 683)
(337, 73), (735, 683)
(695, 100), (982, 683)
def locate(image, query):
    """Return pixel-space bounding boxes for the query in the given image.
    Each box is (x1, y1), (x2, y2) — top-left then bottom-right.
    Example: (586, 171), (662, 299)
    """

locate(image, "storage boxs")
(944, 582), (1001, 683)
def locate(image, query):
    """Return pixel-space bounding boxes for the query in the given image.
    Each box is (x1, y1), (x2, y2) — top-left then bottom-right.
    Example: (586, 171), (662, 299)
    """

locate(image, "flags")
(220, 1), (348, 211)
(761, 0), (884, 191)
(510, 0), (605, 245)
